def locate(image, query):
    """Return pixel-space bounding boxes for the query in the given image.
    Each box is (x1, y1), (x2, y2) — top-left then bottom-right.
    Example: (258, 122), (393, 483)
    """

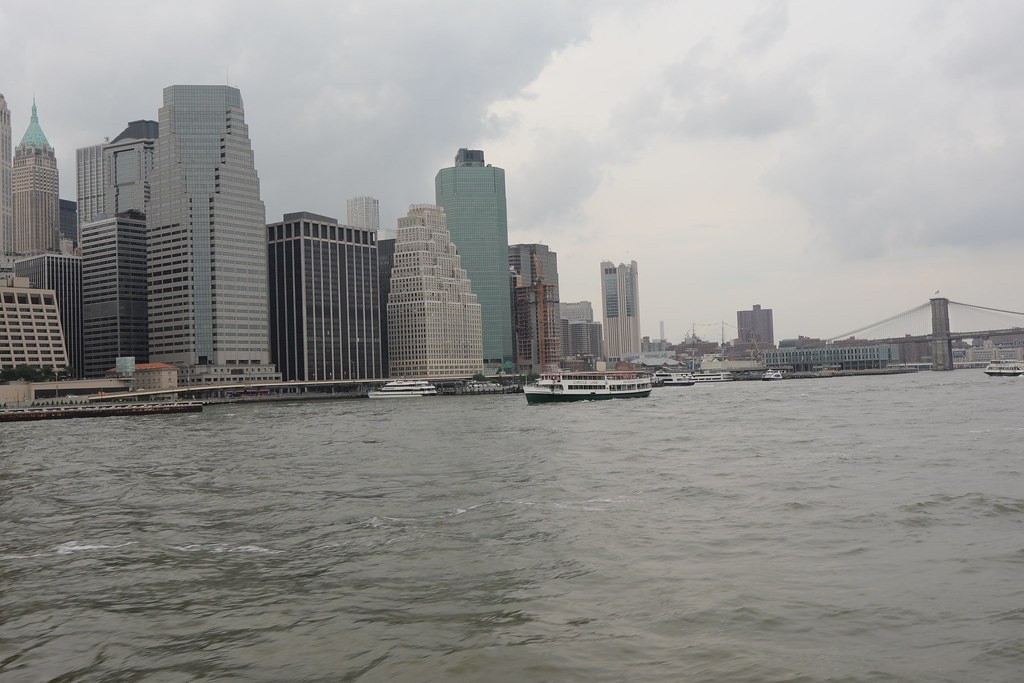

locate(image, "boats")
(522, 366), (653, 406)
(654, 371), (696, 387)
(368, 379), (437, 399)
(761, 369), (783, 381)
(984, 363), (1024, 376)
